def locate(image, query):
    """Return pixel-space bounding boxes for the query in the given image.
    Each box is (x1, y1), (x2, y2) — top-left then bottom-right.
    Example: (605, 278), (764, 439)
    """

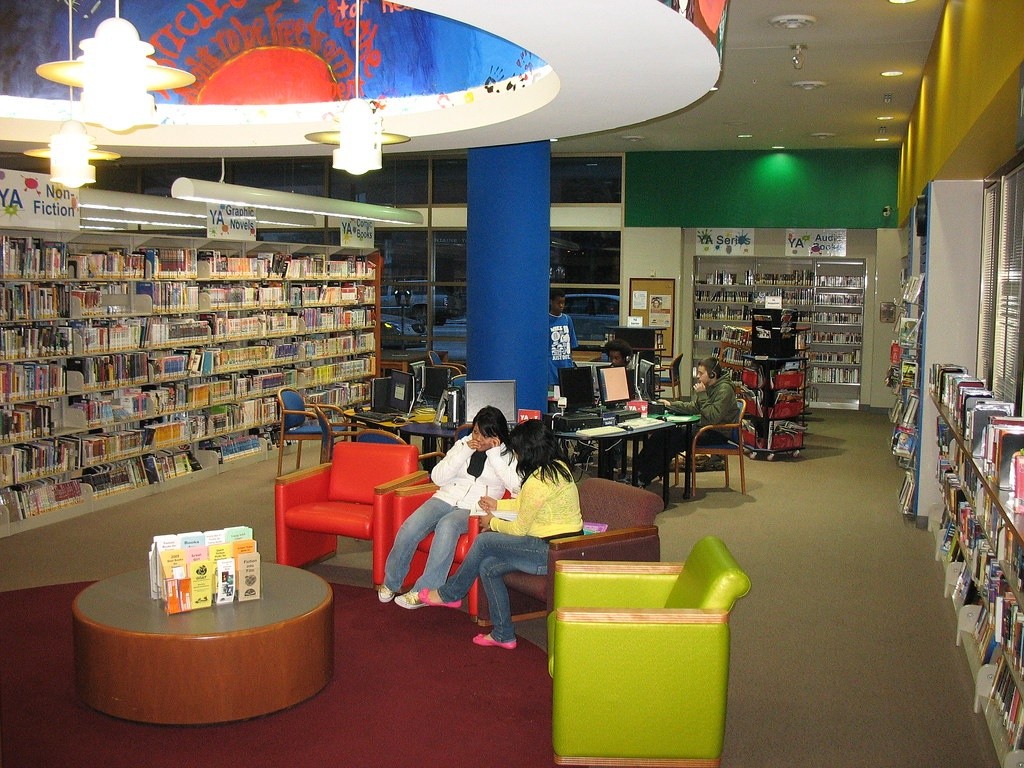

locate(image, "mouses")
(656, 416), (667, 422)
(621, 425), (633, 431)
(394, 418), (405, 423)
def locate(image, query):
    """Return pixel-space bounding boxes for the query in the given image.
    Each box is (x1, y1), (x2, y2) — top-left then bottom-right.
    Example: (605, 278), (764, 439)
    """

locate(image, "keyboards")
(576, 426), (627, 437)
(356, 412), (396, 421)
(618, 415), (665, 430)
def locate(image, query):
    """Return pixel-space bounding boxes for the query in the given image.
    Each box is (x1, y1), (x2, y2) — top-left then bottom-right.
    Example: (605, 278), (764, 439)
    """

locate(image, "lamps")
(36, 0), (197, 133)
(790, 42), (809, 70)
(78, 186), (318, 230)
(170, 157), (424, 226)
(305, 0), (410, 175)
(881, 205), (894, 217)
(24, 0), (120, 190)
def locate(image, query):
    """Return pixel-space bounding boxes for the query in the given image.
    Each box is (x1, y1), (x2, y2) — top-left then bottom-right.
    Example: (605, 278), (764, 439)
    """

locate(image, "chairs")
(674, 399), (747, 498)
(653, 353), (684, 401)
(274, 442), (430, 593)
(454, 424), (474, 442)
(277, 387), (346, 475)
(477, 477), (665, 655)
(546, 534), (753, 768)
(357, 428), (445, 469)
(428, 351), (466, 376)
(391, 481), (512, 622)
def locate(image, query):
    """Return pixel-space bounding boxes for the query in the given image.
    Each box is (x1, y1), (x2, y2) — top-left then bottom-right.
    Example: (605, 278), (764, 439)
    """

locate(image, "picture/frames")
(880, 302), (896, 322)
(628, 277), (676, 358)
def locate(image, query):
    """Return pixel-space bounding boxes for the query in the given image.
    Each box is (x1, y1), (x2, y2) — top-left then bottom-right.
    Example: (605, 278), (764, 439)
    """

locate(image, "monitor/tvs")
(389, 360), (426, 419)
(465, 380), (516, 431)
(559, 359), (655, 418)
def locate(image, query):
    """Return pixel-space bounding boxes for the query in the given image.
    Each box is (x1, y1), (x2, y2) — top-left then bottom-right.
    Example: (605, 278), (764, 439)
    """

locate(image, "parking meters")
(394, 290), (412, 354)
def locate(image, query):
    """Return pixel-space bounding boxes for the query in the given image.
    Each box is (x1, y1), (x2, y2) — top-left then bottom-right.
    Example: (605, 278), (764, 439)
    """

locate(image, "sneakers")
(378, 584), (395, 603)
(395, 589), (430, 609)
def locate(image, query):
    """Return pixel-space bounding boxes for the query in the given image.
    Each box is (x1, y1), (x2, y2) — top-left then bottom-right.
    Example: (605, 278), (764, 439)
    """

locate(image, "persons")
(377, 406), (528, 609)
(418, 419), (583, 648)
(547, 290), (579, 390)
(571, 339), (648, 469)
(618, 357), (739, 492)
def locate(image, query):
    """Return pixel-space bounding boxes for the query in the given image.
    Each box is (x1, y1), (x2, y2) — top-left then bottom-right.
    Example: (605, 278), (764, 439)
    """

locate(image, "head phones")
(709, 361), (719, 379)
(626, 350), (631, 361)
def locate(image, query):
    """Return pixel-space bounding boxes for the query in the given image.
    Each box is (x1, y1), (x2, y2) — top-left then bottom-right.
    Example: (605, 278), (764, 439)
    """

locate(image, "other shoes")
(618, 477), (645, 489)
(418, 588), (461, 608)
(473, 634), (516, 649)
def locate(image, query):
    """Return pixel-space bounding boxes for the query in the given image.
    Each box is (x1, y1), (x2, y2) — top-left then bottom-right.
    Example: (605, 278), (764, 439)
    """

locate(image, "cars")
(381, 312), (422, 349)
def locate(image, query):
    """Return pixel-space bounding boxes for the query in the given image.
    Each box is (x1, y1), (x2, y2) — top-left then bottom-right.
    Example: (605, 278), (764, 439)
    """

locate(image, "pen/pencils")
(485, 485), (489, 496)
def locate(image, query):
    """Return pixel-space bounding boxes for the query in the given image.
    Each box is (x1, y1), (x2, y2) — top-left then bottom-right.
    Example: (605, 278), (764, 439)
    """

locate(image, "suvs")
(561, 294), (620, 341)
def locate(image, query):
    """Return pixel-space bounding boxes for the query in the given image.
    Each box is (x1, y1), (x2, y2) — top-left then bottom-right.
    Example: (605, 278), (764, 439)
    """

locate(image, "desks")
(579, 406), (702, 500)
(380, 350), (448, 372)
(403, 424), (471, 438)
(71, 562), (333, 724)
(554, 418), (677, 509)
(345, 399), (406, 427)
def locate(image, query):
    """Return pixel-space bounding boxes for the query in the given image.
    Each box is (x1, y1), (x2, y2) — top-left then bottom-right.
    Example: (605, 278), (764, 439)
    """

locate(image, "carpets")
(0, 581), (601, 768)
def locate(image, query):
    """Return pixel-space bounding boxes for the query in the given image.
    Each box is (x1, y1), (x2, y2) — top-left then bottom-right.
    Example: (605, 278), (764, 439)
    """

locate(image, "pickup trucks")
(381, 282), (450, 326)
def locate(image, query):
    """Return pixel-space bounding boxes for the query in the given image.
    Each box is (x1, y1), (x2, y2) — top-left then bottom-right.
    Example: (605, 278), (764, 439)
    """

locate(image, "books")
(693, 269), (860, 384)
(0, 234), (375, 522)
(885, 275), (1024, 753)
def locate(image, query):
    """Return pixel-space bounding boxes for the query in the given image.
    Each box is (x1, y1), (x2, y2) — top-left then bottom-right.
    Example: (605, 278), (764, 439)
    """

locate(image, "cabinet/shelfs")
(0, 228), (383, 538)
(741, 353), (807, 459)
(689, 255), (866, 410)
(718, 326), (811, 417)
(927, 389), (1024, 768)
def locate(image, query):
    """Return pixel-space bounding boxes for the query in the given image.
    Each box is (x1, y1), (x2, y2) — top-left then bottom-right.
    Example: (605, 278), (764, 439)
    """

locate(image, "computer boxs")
(542, 402), (665, 432)
(370, 377), (397, 413)
(425, 367), (452, 401)
(446, 386), (462, 429)
